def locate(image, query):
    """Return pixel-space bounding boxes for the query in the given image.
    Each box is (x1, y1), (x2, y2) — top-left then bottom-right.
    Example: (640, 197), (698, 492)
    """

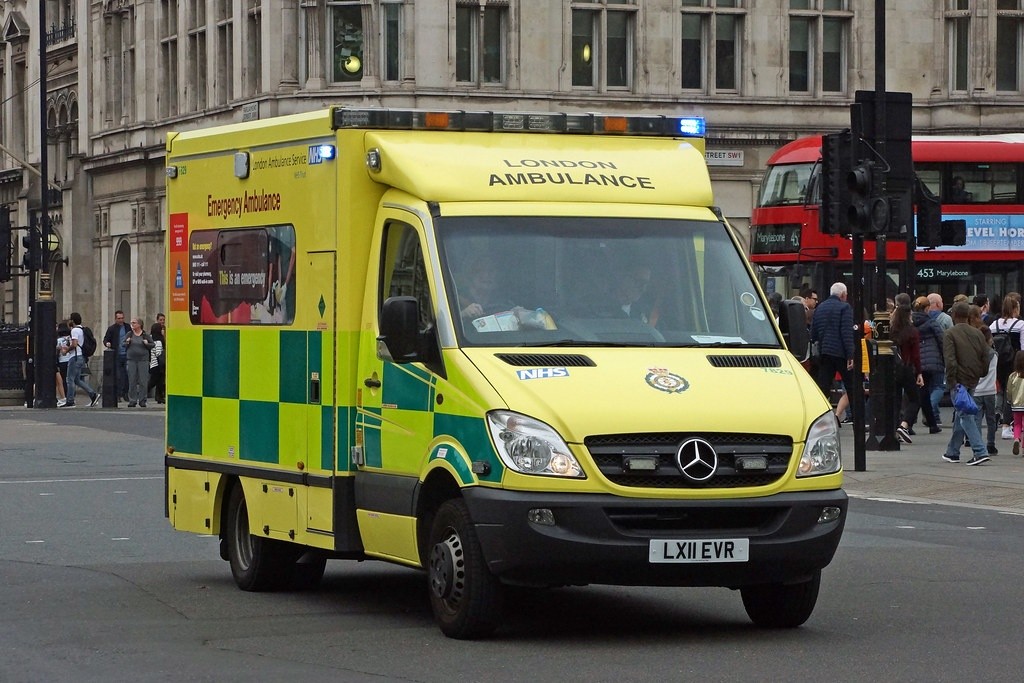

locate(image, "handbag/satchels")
(954, 380), (978, 415)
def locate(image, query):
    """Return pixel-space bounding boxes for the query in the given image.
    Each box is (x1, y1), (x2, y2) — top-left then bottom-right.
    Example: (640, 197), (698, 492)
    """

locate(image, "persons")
(771, 283), (1024, 465)
(951, 175), (968, 205)
(22, 312), (166, 407)
(597, 273), (665, 330)
(449, 254), (528, 323)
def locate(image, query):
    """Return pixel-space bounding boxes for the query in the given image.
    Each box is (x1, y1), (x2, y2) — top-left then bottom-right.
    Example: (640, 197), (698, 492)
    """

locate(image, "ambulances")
(161, 106), (849, 640)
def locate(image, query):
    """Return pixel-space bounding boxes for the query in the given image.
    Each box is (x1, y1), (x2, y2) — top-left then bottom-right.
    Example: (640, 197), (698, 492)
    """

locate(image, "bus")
(751, 133), (1024, 413)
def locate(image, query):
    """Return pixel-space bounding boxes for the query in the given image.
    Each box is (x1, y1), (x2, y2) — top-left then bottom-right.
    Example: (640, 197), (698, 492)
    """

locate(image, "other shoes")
(930, 426), (942, 433)
(57, 392), (165, 408)
(1002, 425), (1014, 439)
(965, 440), (970, 447)
(988, 447), (998, 456)
(994, 413), (1001, 431)
(1012, 438), (1019, 455)
(935, 416), (942, 425)
(921, 418), (928, 427)
(908, 427), (915, 435)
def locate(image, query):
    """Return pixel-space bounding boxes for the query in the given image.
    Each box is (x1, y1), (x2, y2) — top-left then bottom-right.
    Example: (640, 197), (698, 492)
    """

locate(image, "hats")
(953, 294), (968, 303)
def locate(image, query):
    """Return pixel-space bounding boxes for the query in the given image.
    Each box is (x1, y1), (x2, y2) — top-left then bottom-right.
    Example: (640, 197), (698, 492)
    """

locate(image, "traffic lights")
(23, 237), (32, 269)
(844, 161), (891, 236)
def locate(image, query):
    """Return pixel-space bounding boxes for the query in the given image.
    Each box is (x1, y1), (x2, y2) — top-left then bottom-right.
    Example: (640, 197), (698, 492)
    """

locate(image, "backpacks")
(992, 317), (1019, 367)
(70, 327), (96, 357)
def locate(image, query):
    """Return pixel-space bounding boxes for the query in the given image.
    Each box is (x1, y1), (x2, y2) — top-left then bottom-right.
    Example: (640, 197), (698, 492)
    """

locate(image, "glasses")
(810, 298), (819, 302)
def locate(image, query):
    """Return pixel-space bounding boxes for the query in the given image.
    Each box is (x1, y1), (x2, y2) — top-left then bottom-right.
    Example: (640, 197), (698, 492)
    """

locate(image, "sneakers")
(941, 453), (960, 463)
(965, 455), (990, 466)
(897, 426), (912, 443)
(840, 416), (854, 425)
